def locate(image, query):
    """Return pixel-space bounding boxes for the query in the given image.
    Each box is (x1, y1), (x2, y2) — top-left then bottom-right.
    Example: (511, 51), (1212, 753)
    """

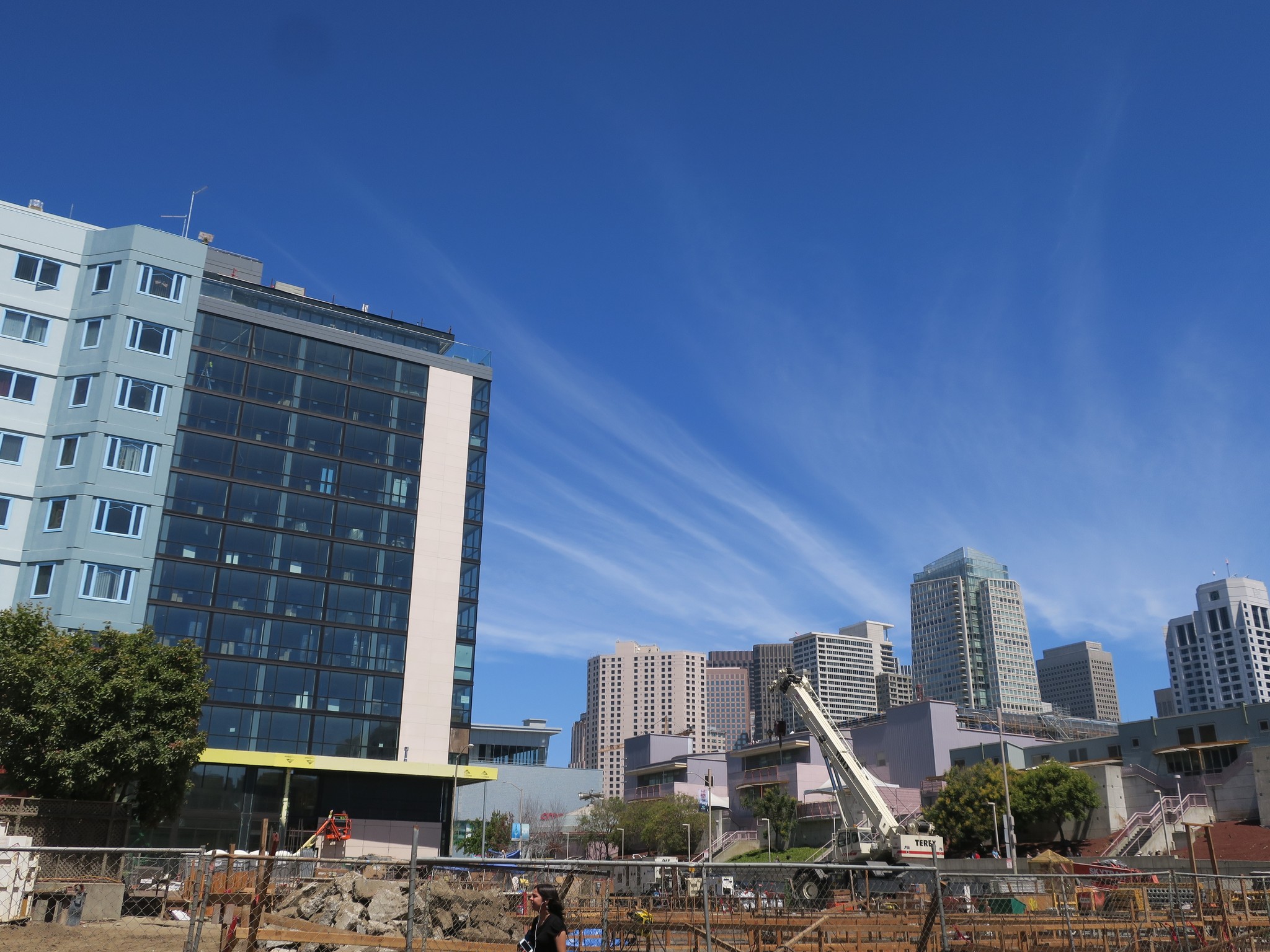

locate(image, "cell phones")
(519, 939), (533, 952)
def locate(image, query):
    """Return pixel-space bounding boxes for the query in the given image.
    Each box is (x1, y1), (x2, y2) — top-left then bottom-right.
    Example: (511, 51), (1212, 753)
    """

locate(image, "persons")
(1066, 847), (1074, 857)
(1035, 849), (1040, 855)
(1027, 852), (1031, 858)
(699, 790), (708, 802)
(992, 847), (1001, 859)
(971, 850), (980, 859)
(520, 884), (567, 952)
(1137, 851), (1142, 856)
(1148, 850), (1153, 856)
(1155, 849), (1161, 856)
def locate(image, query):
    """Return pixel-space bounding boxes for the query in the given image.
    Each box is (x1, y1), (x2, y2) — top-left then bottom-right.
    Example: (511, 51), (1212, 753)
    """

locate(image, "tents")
(1027, 849), (1070, 892)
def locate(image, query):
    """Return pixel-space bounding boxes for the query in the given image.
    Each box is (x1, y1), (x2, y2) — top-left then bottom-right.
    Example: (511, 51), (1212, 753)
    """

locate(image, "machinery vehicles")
(765, 666), (946, 913)
(1039, 858), (1161, 914)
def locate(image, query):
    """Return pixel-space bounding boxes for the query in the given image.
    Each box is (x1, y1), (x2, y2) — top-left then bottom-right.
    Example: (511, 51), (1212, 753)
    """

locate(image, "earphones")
(542, 900), (546, 904)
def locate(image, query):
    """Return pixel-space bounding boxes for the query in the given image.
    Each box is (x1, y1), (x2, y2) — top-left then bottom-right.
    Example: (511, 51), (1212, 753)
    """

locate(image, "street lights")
(1146, 789), (1171, 856)
(715, 820), (719, 846)
(562, 832), (569, 860)
(761, 818), (771, 862)
(980, 802), (1000, 852)
(448, 743), (474, 856)
(1173, 774), (1187, 831)
(616, 828), (624, 859)
(683, 823), (691, 862)
(502, 781), (523, 858)
(686, 767), (713, 863)
(972, 707), (1019, 874)
